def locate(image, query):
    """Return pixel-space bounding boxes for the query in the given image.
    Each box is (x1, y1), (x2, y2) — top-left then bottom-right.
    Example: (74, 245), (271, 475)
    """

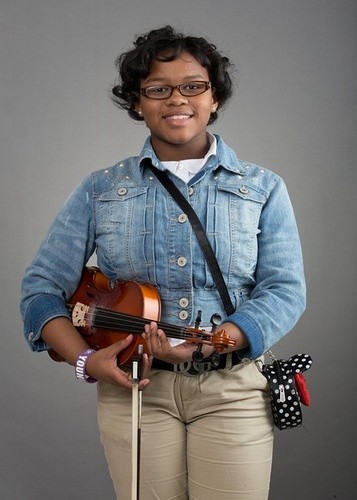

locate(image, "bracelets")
(75, 348), (98, 383)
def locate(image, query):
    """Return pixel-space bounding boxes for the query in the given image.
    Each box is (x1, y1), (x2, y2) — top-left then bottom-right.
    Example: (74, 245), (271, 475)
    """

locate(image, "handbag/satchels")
(263, 353), (313, 430)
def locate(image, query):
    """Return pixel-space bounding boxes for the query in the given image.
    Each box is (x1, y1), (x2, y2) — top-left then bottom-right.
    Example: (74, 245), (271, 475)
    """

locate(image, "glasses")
(140, 81), (211, 100)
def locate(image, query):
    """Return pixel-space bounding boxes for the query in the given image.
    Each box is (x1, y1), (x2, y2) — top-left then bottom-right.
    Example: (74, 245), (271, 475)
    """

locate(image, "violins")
(46, 265), (237, 369)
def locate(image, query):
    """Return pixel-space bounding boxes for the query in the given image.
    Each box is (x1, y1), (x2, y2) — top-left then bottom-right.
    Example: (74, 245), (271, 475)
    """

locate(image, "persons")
(19, 25), (306, 500)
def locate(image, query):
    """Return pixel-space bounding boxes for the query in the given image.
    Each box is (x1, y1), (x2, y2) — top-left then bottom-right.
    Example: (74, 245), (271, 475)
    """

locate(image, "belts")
(150, 348), (246, 377)
